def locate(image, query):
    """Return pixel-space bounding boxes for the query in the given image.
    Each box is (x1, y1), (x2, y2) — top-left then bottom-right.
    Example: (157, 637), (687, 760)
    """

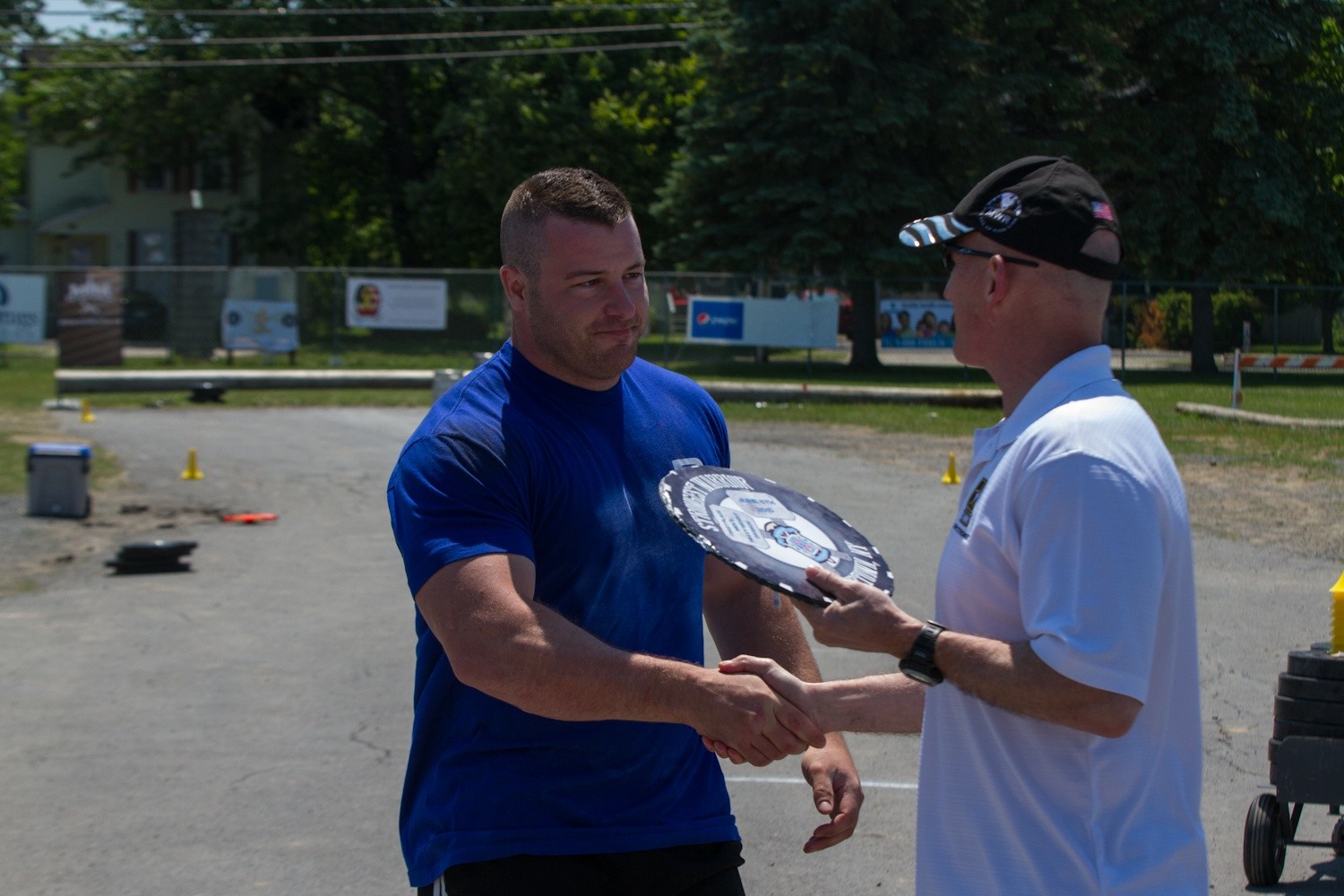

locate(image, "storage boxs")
(24, 444), (92, 520)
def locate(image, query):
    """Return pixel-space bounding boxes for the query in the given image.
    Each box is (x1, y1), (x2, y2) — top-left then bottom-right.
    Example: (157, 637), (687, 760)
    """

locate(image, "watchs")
(900, 616), (946, 685)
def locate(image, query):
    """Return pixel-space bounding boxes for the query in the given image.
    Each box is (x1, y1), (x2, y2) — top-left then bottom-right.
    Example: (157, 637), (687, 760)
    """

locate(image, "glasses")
(940, 242), (1039, 274)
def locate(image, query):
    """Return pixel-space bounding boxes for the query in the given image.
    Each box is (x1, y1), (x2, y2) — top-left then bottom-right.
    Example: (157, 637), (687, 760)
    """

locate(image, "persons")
(386, 167), (866, 895)
(880, 310), (949, 339)
(782, 156), (1209, 896)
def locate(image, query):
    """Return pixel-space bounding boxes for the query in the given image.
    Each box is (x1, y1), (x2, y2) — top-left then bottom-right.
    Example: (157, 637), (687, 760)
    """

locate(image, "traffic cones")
(181, 447), (203, 480)
(80, 398), (96, 424)
(940, 452), (960, 485)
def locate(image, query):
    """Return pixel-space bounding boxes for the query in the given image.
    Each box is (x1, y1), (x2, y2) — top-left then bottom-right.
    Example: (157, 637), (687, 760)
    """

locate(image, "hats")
(898, 154), (1125, 281)
